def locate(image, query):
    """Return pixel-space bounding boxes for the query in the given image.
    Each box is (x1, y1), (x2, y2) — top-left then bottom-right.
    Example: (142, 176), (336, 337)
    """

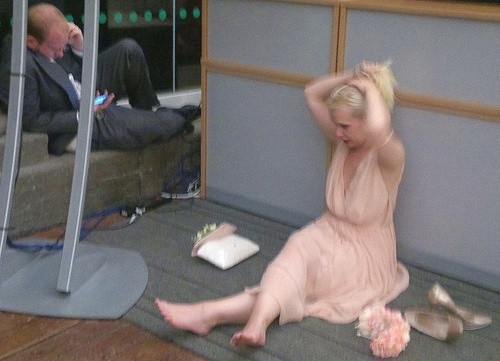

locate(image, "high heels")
(402, 308), (464, 343)
(426, 281), (492, 332)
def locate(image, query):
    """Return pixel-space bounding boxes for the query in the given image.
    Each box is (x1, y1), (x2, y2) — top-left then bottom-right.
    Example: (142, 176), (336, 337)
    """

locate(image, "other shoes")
(157, 104), (201, 122)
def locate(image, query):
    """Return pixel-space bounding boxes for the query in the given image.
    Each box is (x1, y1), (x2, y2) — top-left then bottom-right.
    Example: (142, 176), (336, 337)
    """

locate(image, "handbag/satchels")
(191, 221), (260, 270)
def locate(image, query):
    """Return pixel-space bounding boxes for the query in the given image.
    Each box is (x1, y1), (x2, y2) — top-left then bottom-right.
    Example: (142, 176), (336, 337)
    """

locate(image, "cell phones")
(95, 95), (108, 104)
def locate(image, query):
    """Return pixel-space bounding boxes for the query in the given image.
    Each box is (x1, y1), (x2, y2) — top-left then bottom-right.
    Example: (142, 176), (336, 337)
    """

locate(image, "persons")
(0, 3), (202, 157)
(156, 61), (405, 348)
